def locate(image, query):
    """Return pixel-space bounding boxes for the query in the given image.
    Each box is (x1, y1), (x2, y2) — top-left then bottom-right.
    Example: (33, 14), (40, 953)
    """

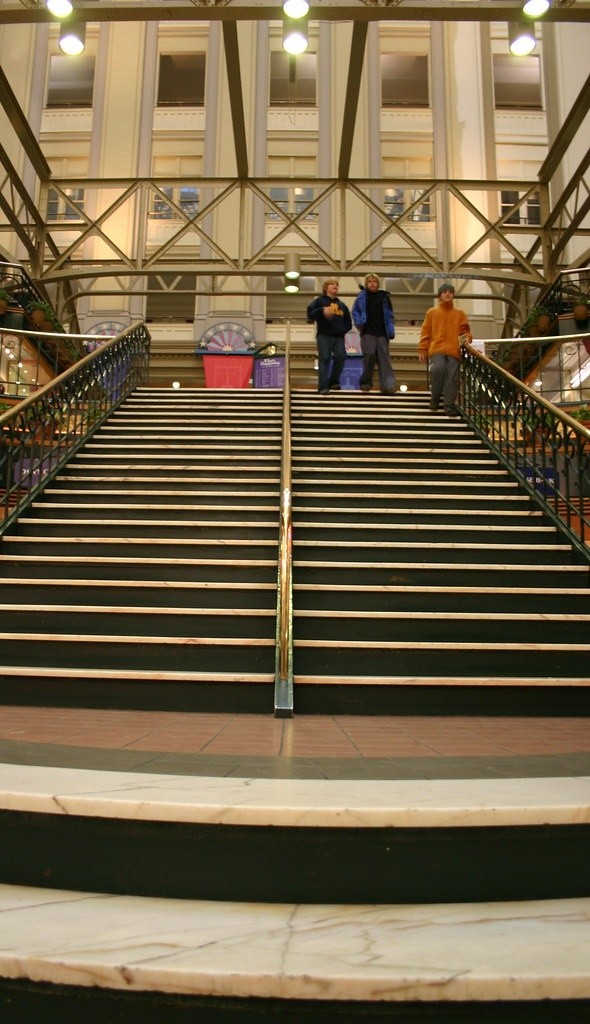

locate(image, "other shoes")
(382, 386), (396, 393)
(430, 402), (438, 410)
(445, 407), (457, 416)
(320, 389), (329, 395)
(331, 383), (341, 390)
(361, 383), (371, 391)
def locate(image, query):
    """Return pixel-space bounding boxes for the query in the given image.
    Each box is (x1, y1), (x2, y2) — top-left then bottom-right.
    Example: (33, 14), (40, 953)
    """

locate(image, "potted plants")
(26, 404), (66, 440)
(572, 296), (589, 325)
(568, 406), (590, 445)
(521, 414), (556, 447)
(0, 290), (10, 316)
(495, 306), (553, 372)
(26, 300), (79, 370)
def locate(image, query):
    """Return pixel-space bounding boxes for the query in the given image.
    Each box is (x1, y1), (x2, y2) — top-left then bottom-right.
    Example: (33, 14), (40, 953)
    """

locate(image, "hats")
(438, 282), (454, 296)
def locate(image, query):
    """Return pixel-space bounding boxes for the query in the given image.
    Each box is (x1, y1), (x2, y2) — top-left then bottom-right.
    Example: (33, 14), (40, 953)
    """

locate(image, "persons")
(351, 273), (397, 394)
(417, 283), (473, 416)
(306, 279), (352, 395)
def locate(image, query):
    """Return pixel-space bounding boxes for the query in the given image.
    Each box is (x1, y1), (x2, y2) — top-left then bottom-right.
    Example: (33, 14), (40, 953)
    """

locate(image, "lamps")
(520, 0), (551, 20)
(570, 362), (590, 389)
(47, 0), (74, 19)
(283, 252), (302, 295)
(508, 21), (536, 57)
(282, 0), (310, 56)
(59, 20), (86, 56)
